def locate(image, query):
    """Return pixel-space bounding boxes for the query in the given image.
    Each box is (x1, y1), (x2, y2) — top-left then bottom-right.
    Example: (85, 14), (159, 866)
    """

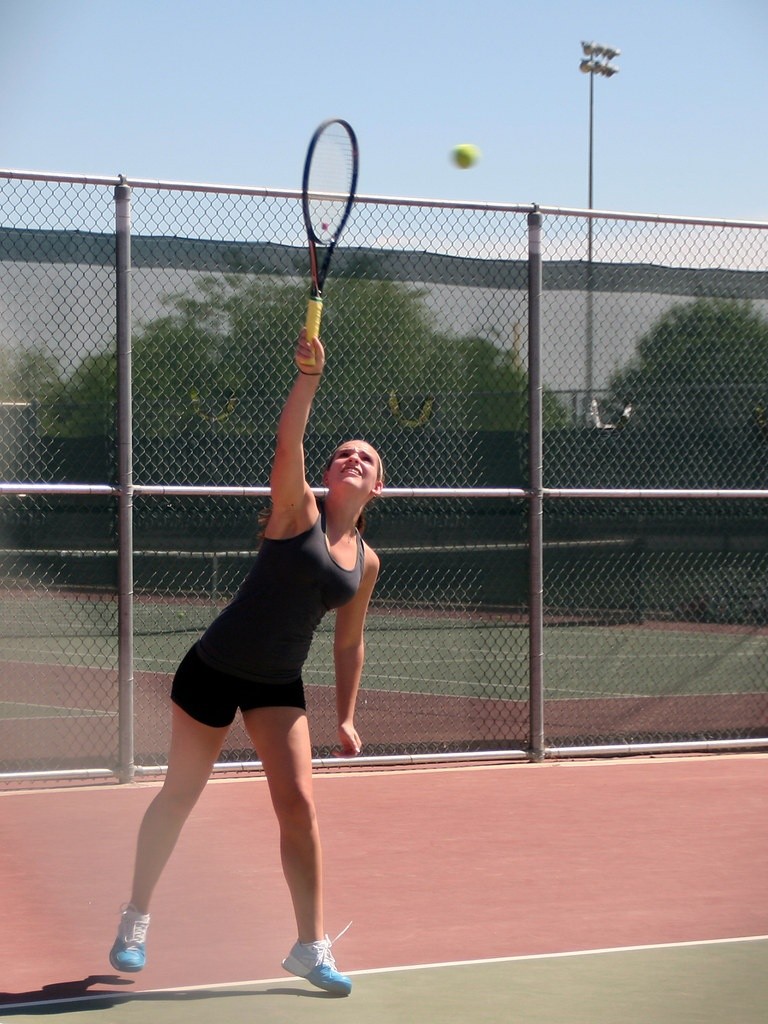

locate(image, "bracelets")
(299, 369), (322, 375)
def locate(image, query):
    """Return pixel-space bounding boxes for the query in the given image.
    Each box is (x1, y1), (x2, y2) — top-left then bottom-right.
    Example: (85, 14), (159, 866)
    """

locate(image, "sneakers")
(281, 920), (354, 994)
(110, 902), (151, 973)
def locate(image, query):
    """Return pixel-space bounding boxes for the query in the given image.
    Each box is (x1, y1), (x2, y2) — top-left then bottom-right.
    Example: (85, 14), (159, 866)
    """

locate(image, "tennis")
(452, 144), (478, 169)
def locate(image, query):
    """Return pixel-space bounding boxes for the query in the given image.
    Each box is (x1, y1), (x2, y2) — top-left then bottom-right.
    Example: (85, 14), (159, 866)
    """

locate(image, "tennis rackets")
(297, 118), (360, 367)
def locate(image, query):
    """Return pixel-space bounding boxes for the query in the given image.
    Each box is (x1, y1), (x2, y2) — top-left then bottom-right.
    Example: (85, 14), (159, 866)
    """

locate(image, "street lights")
(578, 39), (622, 423)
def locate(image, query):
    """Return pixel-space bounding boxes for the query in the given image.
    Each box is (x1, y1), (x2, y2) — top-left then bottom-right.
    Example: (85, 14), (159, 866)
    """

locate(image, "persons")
(107, 326), (383, 996)
